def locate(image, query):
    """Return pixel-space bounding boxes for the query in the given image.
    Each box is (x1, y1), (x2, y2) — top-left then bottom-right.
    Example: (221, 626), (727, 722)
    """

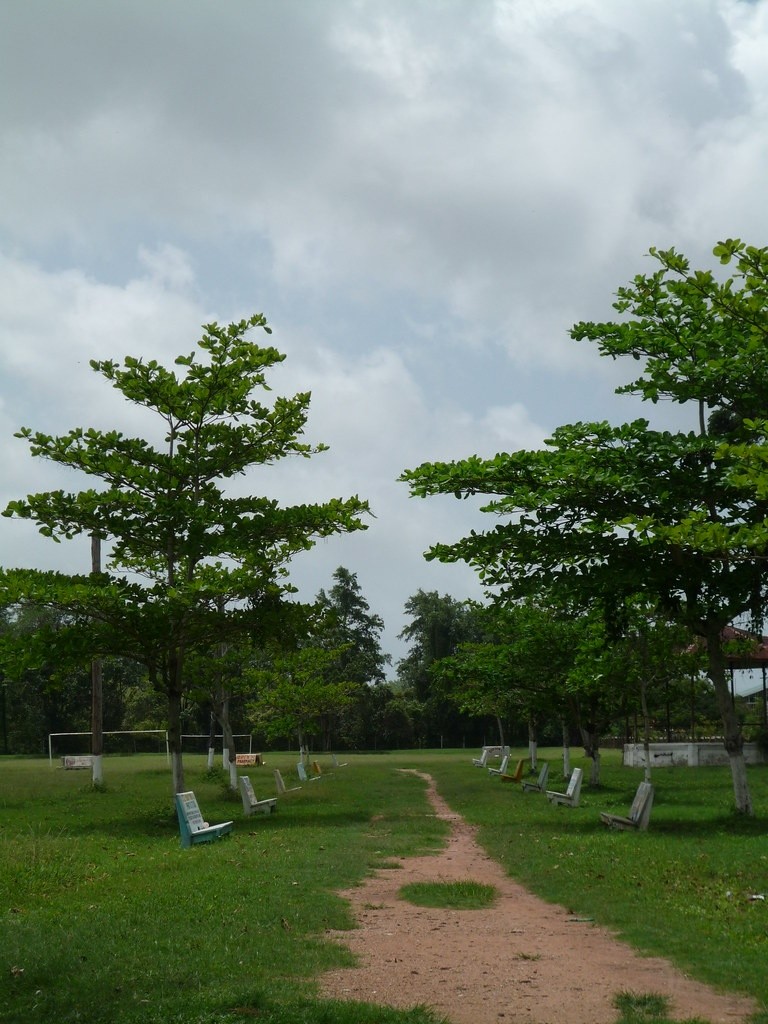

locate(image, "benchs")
(546, 768), (583, 807)
(598, 781), (654, 830)
(489, 756), (507, 777)
(273, 770), (301, 795)
(481, 746), (511, 759)
(501, 760), (522, 783)
(297, 754), (348, 783)
(236, 753), (262, 766)
(64, 755), (94, 769)
(174, 791), (235, 848)
(239, 776), (276, 815)
(473, 750), (488, 767)
(520, 764), (549, 791)
